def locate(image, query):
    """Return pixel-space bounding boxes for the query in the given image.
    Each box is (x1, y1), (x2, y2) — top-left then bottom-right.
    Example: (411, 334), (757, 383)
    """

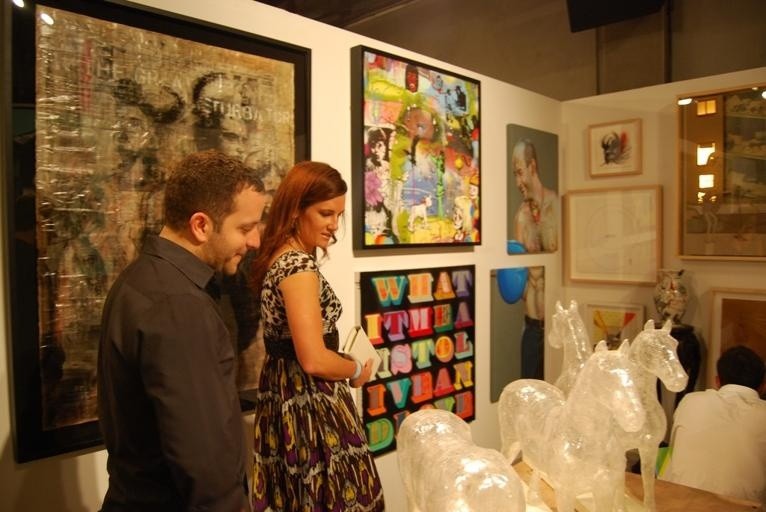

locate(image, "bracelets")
(350, 358), (361, 380)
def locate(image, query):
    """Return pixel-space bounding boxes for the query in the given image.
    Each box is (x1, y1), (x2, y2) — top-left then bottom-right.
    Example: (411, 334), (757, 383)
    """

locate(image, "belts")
(523, 314), (544, 331)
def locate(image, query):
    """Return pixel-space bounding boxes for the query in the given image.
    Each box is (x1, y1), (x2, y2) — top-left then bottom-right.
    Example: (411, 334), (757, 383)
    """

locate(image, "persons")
(653, 343), (765, 506)
(509, 137), (557, 383)
(195, 100), (252, 163)
(96, 148), (267, 512)
(248, 159), (390, 512)
(56, 94), (175, 317)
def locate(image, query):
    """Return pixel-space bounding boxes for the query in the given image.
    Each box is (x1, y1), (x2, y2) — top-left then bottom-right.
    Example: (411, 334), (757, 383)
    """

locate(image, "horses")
(395, 299), (692, 512)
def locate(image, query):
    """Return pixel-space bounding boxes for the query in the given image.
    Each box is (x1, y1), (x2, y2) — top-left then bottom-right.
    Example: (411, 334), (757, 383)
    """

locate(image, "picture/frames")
(672, 84), (765, 263)
(564, 184), (662, 287)
(582, 300), (647, 352)
(706, 283), (766, 390)
(3, 3), (313, 457)
(586, 117), (645, 179)
